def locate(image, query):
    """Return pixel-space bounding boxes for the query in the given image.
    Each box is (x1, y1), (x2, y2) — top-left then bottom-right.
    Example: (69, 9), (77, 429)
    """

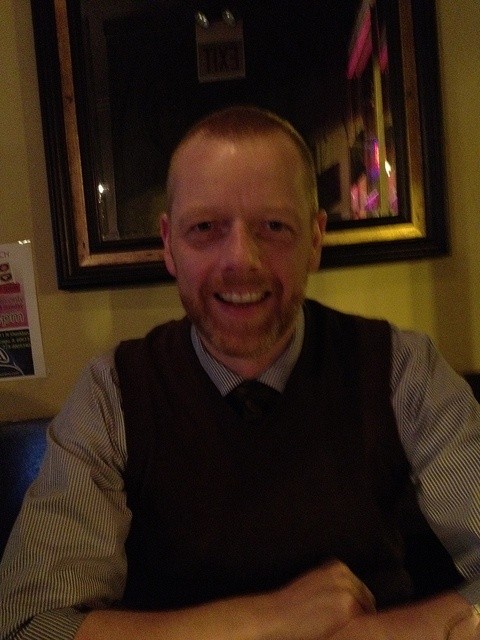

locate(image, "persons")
(1, 107), (479, 640)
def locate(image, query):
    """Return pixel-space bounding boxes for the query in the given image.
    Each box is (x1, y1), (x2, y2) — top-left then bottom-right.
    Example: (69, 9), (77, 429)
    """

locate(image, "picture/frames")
(29, 0), (454, 291)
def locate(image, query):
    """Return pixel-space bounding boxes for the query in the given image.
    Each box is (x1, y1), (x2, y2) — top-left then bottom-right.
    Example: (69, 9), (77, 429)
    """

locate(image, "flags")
(343, 0), (377, 78)
